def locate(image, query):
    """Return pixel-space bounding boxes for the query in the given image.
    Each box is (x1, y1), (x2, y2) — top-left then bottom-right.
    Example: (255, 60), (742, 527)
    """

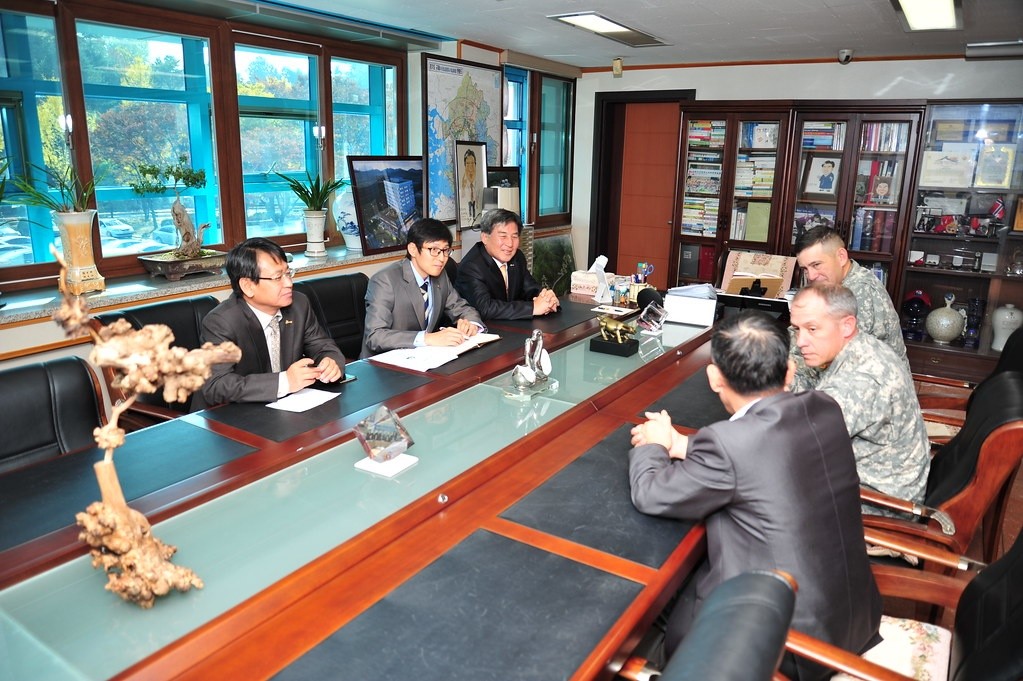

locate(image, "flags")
(990, 195), (1005, 219)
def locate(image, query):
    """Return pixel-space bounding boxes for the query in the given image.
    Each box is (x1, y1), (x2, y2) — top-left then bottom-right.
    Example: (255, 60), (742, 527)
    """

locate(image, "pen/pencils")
(439, 327), (469, 340)
(302, 354), (307, 367)
(631, 273), (647, 283)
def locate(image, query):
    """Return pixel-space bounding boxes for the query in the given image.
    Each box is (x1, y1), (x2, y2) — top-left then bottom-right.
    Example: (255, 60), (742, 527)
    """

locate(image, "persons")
(190, 238), (346, 413)
(359, 218), (487, 360)
(783, 281), (931, 522)
(628, 309), (883, 681)
(455, 209), (560, 320)
(785, 226), (911, 375)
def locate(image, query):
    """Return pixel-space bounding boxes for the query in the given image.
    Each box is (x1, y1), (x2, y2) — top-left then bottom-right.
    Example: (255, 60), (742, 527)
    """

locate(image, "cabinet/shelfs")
(663, 102), (790, 297)
(775, 104), (927, 311)
(887, 97), (1022, 389)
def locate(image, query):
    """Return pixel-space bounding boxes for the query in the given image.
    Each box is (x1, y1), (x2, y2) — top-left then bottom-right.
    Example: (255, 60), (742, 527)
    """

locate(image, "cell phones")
(339, 374), (357, 383)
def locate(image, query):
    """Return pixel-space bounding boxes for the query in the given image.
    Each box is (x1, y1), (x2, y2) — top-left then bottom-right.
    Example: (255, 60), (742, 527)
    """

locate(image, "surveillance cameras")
(839, 49), (854, 64)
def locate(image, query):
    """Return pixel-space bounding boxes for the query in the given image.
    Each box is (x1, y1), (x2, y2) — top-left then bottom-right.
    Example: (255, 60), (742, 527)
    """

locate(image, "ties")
(419, 283), (429, 331)
(268, 316), (281, 373)
(501, 265), (508, 293)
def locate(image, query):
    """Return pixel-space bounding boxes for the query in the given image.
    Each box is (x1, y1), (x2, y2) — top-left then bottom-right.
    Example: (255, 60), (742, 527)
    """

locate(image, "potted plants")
(273, 169), (350, 257)
(126, 156), (230, 281)
(2, 154), (109, 296)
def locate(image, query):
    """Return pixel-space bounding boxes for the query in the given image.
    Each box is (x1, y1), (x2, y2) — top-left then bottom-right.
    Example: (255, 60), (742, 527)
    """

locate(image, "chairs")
(611, 326), (1023, 681)
(90, 297), (220, 421)
(1, 354), (114, 474)
(292, 271), (370, 363)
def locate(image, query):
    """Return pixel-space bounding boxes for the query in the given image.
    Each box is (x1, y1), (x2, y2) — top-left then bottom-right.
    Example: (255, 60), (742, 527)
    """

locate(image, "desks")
(0, 291), (786, 681)
(1, 295), (731, 680)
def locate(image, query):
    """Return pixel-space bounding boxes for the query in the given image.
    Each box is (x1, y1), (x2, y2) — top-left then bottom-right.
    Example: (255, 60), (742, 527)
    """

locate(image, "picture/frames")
(452, 139), (488, 231)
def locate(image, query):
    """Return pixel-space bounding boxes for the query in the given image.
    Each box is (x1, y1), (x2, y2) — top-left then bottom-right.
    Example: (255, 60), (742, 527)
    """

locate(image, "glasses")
(417, 244), (454, 257)
(250, 267), (296, 283)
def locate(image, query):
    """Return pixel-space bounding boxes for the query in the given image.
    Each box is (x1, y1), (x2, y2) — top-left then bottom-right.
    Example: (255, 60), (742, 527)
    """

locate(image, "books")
(792, 121), (912, 253)
(680, 119), (779, 243)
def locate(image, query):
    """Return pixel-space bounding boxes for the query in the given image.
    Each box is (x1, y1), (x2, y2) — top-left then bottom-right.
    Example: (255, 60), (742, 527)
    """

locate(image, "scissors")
(642, 262), (654, 276)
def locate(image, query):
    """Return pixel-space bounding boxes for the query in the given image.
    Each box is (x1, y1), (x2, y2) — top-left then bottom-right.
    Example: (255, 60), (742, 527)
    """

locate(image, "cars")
(0, 219), (63, 267)
(101, 237), (168, 257)
(152, 224), (182, 246)
(98, 217), (135, 238)
